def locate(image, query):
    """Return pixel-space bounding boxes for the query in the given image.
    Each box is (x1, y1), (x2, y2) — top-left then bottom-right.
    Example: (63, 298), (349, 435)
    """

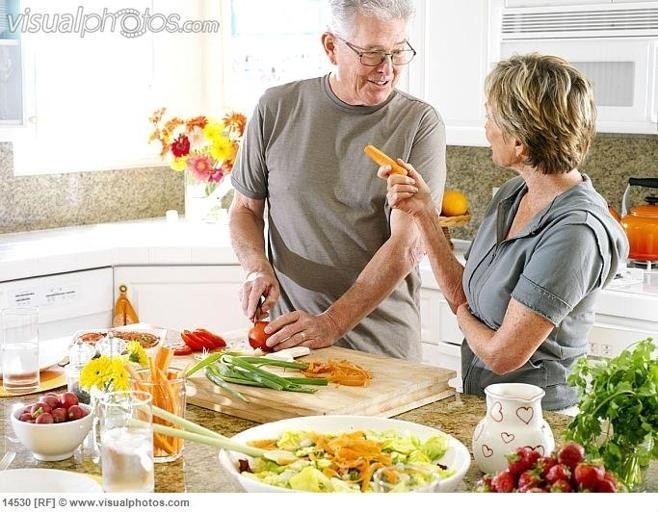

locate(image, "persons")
(229, 1), (446, 362)
(376, 52), (630, 417)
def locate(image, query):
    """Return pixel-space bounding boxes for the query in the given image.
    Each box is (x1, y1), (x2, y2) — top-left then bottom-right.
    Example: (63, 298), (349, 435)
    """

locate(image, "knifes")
(253, 297), (263, 324)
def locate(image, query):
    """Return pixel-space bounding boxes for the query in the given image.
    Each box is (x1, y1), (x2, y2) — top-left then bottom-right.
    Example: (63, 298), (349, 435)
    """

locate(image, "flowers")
(147, 107), (247, 198)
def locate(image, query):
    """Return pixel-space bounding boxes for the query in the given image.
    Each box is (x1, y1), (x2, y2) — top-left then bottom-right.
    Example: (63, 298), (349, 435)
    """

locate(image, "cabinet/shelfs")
(114, 265), (250, 335)
(420, 288), (658, 439)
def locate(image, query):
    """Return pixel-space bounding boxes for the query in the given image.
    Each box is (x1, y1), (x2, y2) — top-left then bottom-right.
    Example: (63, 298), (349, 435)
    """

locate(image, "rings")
(300, 331), (307, 341)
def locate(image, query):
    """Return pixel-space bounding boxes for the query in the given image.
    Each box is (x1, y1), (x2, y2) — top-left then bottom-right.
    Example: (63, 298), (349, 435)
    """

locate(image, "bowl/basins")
(11, 405), (94, 461)
(220, 415), (472, 493)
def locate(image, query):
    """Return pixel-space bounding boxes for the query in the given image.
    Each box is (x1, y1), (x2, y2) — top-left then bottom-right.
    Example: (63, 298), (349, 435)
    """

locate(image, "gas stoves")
(593, 261), (658, 321)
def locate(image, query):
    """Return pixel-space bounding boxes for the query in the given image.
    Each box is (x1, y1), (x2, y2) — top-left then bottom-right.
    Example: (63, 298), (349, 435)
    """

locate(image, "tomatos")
(248, 321), (282, 353)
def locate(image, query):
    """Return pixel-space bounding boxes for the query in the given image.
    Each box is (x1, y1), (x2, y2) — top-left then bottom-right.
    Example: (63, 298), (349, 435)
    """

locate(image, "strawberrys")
(475, 444), (616, 493)
(21, 392), (85, 424)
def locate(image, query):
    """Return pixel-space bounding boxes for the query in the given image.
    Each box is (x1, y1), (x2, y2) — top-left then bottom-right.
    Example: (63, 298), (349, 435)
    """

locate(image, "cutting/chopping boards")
(179, 345), (456, 422)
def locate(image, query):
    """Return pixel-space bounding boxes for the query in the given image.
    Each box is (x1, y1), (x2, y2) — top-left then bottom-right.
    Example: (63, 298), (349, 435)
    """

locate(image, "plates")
(474, 474), (628, 494)
(69, 325), (166, 357)
(1, 469), (102, 492)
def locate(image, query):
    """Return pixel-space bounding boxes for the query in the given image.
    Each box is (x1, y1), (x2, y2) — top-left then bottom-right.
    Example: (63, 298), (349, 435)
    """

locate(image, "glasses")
(337, 37), (418, 67)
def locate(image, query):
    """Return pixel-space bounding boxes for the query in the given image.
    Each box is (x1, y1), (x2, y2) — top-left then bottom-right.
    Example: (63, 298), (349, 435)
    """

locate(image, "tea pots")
(607, 178), (658, 260)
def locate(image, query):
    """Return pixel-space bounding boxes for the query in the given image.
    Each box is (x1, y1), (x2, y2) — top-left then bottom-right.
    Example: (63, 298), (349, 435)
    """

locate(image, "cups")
(88, 367), (186, 492)
(63, 331), (128, 406)
(1, 305), (41, 392)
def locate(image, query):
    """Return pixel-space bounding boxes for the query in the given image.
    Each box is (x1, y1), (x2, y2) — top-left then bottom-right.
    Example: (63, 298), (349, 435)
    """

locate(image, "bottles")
(219, 209), (229, 224)
(166, 210), (180, 232)
(472, 383), (556, 475)
